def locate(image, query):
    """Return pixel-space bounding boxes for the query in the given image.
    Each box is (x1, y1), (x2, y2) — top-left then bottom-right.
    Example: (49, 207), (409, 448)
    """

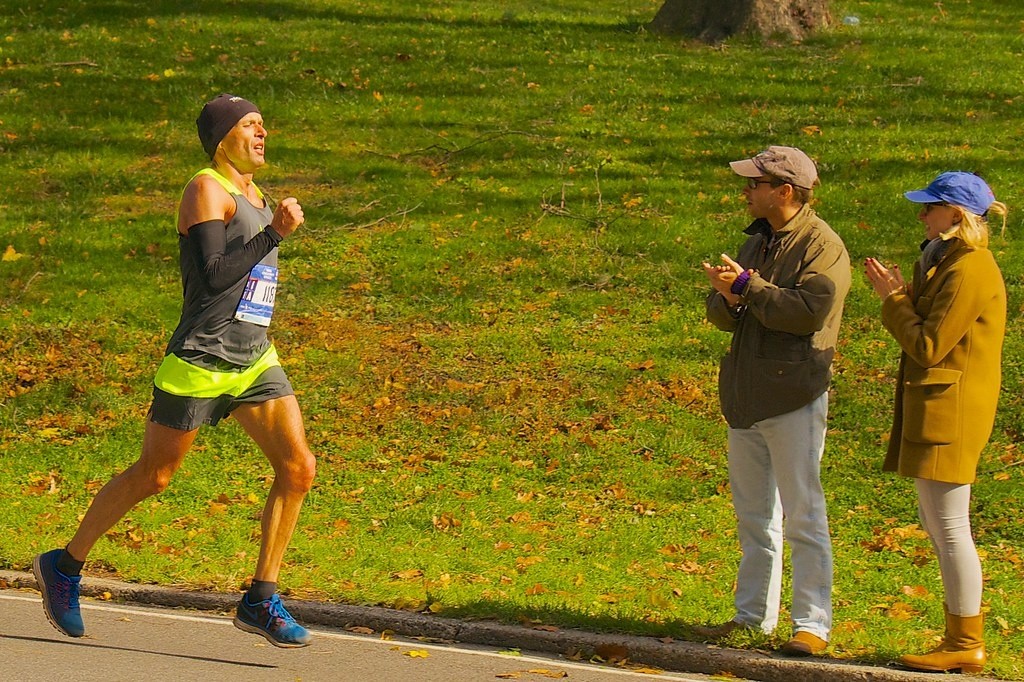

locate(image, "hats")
(196, 94), (261, 161)
(903, 171), (996, 216)
(729, 146), (818, 189)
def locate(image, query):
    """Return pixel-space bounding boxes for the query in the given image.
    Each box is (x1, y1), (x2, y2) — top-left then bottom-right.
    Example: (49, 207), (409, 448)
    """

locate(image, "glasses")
(746, 177), (771, 189)
(923, 202), (948, 213)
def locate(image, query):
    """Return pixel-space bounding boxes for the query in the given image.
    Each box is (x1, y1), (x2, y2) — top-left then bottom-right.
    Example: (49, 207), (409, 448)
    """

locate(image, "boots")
(899, 601), (986, 674)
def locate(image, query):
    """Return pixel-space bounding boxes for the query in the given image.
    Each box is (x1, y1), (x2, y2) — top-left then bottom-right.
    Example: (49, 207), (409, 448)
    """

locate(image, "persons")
(702, 146), (852, 655)
(32, 94), (315, 648)
(862, 172), (1007, 674)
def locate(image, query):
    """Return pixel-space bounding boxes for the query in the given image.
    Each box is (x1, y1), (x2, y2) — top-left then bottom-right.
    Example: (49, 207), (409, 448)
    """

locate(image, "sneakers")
(690, 619), (750, 639)
(783, 631), (827, 656)
(233, 592), (314, 648)
(31, 548), (84, 638)
